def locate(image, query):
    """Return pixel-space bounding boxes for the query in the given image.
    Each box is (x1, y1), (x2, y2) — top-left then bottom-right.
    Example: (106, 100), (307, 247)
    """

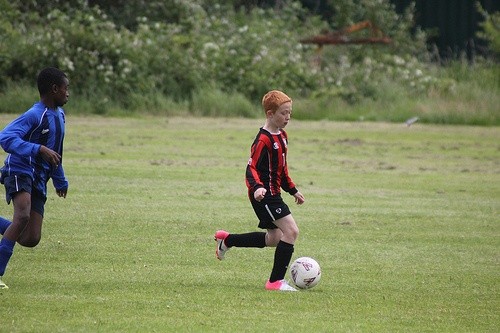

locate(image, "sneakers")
(265, 277), (298, 291)
(214, 229), (230, 260)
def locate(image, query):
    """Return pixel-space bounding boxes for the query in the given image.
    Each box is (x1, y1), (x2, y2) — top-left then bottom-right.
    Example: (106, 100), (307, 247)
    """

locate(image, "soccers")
(290, 256), (321, 289)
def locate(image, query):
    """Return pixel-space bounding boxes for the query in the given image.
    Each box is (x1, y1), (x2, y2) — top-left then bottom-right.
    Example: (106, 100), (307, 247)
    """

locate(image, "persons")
(216, 90), (304, 291)
(0, 66), (70, 290)
